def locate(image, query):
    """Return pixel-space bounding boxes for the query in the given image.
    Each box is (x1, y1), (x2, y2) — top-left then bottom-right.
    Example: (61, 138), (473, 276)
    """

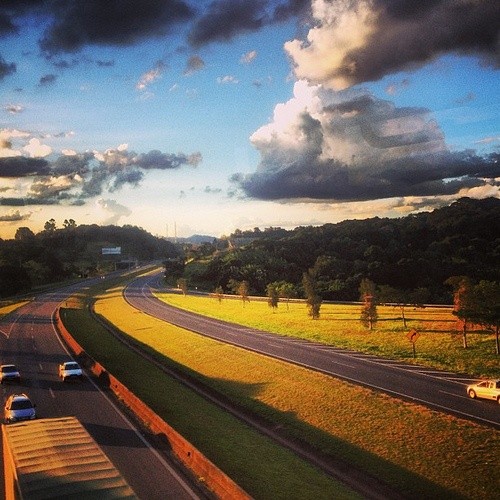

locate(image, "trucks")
(1, 416), (139, 500)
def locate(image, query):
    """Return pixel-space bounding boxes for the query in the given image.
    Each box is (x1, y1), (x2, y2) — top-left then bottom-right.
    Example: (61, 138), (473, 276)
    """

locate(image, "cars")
(58, 362), (83, 382)
(6, 393), (36, 423)
(0, 365), (21, 382)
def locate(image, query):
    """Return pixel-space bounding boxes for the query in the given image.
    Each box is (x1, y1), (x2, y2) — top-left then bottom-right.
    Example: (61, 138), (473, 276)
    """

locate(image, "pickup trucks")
(466, 379), (500, 404)
